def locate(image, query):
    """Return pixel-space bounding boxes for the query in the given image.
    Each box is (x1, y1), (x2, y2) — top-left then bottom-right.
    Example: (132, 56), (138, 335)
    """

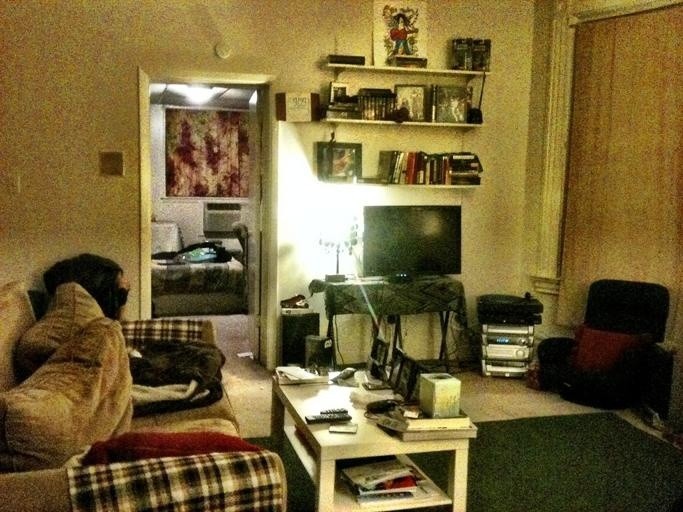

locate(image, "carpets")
(244, 411), (682, 512)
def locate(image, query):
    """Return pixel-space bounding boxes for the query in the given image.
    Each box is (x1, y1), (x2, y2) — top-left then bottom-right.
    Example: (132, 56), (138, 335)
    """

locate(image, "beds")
(152, 256), (249, 318)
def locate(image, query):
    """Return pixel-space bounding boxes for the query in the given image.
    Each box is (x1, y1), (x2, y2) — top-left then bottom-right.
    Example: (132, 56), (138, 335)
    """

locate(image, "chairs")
(537, 279), (673, 410)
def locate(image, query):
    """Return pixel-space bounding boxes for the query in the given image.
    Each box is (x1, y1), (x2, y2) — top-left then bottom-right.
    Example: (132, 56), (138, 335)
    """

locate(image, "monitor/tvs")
(363, 205), (461, 276)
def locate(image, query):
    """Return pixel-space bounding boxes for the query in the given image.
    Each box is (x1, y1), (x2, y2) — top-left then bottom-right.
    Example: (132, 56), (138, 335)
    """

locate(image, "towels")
(62, 448), (284, 512)
(120, 321), (203, 348)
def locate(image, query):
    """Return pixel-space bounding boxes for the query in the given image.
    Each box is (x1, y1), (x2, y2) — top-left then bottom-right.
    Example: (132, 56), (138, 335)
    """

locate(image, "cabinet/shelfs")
(309, 275), (466, 374)
(320, 62), (492, 188)
(151, 220), (182, 255)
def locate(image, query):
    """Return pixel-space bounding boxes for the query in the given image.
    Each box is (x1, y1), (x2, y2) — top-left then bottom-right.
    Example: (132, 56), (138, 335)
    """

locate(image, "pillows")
(0, 280), (38, 394)
(82, 431), (261, 466)
(574, 324), (655, 378)
(11, 281), (105, 386)
(0, 317), (132, 471)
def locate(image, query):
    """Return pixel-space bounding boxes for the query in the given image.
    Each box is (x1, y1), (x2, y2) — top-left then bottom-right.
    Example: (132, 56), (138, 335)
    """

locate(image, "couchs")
(0, 320), (289, 512)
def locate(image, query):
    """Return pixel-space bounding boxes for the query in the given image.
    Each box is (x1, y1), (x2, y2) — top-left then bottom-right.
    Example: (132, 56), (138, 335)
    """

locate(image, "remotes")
(320, 408), (348, 414)
(305, 413), (352, 424)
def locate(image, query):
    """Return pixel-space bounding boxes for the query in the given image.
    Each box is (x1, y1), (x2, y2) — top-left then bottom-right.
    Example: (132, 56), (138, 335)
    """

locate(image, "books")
(335, 454), (437, 510)
(472, 38), (491, 71)
(385, 52), (427, 68)
(450, 37), (475, 71)
(324, 54), (365, 65)
(274, 366), (328, 386)
(321, 80), (483, 185)
(375, 403), (479, 444)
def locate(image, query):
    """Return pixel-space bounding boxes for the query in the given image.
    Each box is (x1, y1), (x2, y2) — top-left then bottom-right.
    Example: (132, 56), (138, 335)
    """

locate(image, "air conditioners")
(204, 202), (241, 233)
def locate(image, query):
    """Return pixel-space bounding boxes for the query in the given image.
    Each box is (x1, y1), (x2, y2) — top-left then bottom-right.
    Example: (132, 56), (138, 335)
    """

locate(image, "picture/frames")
(394, 84), (426, 122)
(435, 86), (468, 123)
(329, 81), (349, 103)
(316, 141), (362, 184)
